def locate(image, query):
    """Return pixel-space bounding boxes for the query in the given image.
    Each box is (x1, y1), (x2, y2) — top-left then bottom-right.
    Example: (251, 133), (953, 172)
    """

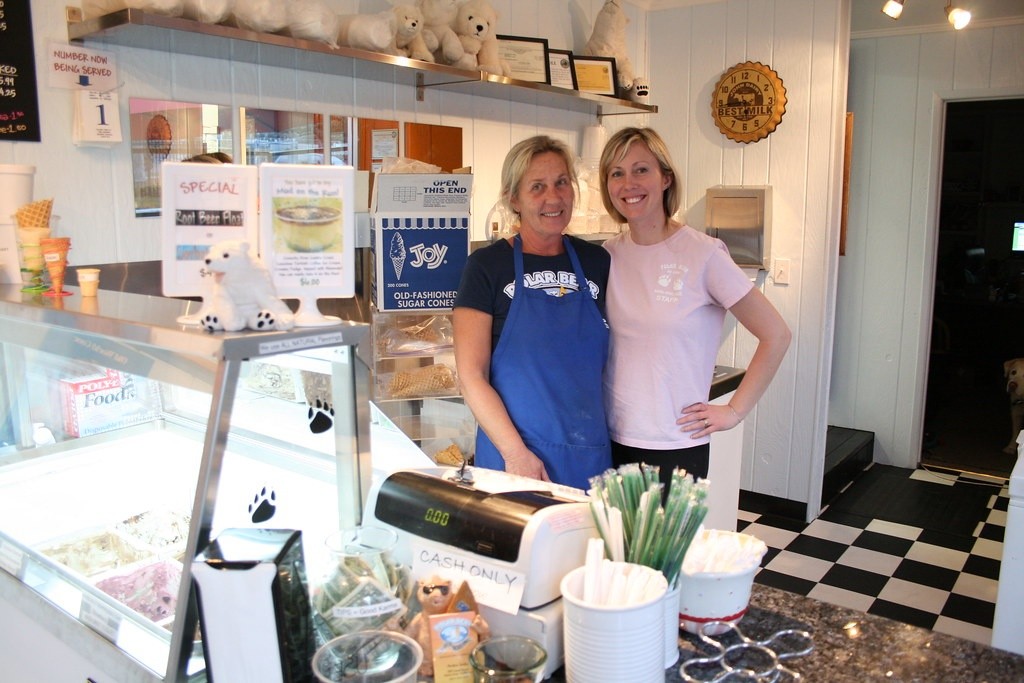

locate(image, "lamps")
(943, 0), (972, 31)
(883, 0), (907, 19)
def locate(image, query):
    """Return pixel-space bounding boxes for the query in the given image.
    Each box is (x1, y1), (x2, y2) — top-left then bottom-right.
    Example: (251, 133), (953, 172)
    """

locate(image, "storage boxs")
(369, 166), (473, 310)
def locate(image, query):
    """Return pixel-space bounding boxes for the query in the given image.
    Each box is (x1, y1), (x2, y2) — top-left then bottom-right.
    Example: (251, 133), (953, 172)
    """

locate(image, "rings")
(703, 420), (708, 428)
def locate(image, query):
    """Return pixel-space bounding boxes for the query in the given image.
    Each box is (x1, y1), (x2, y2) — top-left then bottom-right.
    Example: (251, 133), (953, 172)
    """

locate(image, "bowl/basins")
(678, 528), (767, 636)
(274, 205), (343, 252)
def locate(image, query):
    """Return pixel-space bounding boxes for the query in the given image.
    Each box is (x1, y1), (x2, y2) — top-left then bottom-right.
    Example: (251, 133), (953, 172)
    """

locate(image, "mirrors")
(128, 96), (462, 218)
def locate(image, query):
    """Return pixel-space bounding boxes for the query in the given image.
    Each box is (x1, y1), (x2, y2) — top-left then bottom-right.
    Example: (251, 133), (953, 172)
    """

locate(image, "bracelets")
(729, 405), (742, 423)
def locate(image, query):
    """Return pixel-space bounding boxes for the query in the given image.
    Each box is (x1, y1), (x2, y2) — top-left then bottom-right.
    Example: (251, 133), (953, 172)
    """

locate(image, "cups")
(663, 579), (681, 670)
(559, 561), (668, 683)
(469, 635), (547, 683)
(311, 630), (423, 683)
(80, 296), (99, 316)
(76, 269), (101, 297)
(580, 124), (630, 233)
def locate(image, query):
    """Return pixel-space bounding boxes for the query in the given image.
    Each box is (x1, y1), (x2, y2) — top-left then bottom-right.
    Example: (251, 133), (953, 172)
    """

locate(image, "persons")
(452, 135), (611, 495)
(512, 127), (791, 508)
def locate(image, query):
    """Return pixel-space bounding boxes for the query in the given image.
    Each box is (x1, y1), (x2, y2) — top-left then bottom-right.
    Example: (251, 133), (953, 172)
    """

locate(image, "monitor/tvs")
(1012, 222), (1024, 251)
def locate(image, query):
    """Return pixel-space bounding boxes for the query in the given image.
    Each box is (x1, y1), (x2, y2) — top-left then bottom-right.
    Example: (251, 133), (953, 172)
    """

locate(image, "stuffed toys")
(580, 0), (650, 105)
(200, 240), (295, 332)
(127, 0), (512, 78)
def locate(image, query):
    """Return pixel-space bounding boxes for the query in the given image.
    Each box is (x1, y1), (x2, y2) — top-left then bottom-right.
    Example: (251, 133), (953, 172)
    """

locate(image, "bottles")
(311, 523), (414, 676)
